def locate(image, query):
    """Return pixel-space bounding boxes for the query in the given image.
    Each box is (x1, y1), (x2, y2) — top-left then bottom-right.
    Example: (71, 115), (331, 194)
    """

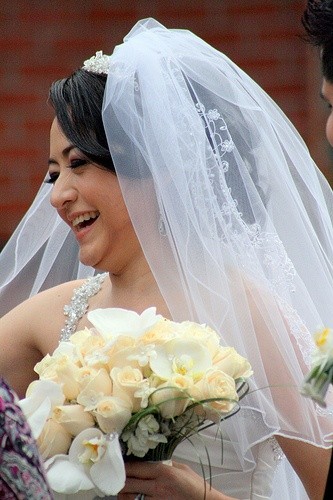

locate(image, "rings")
(134, 494), (145, 500)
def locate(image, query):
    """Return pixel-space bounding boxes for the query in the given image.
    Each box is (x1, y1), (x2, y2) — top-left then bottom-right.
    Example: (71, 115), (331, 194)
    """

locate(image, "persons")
(300, 0), (333, 148)
(0, 18), (333, 500)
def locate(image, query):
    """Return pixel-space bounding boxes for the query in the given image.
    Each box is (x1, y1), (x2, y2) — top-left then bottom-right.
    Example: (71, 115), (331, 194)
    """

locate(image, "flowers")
(300, 329), (333, 409)
(19, 307), (302, 500)
